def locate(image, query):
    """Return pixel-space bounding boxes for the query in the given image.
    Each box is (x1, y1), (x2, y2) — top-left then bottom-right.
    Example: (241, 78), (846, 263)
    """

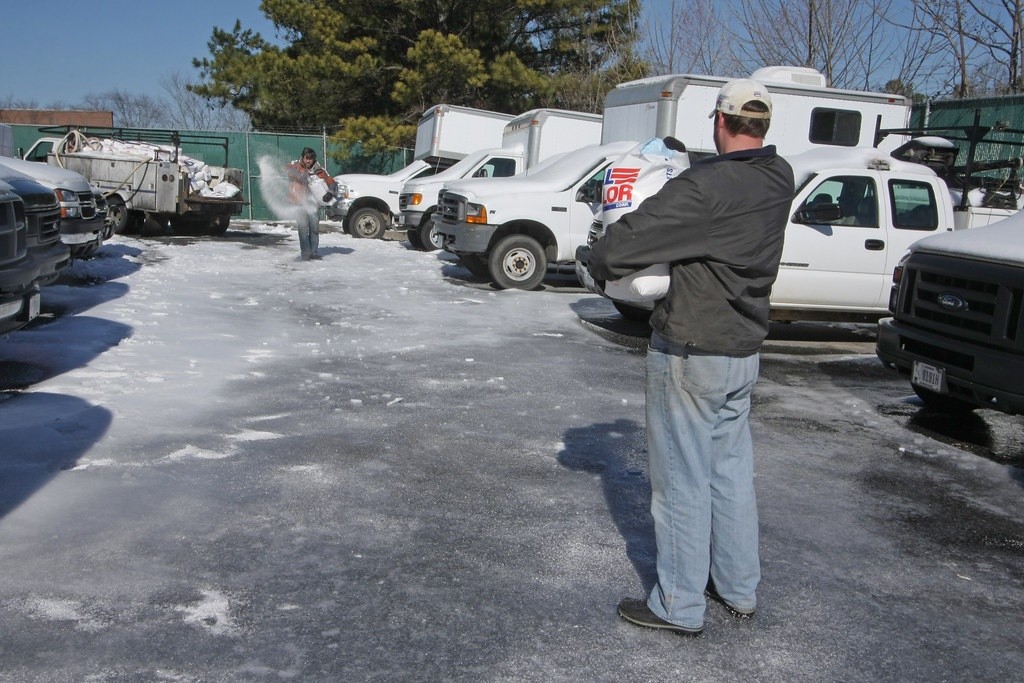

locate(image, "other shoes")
(616, 597), (705, 636)
(703, 572), (757, 619)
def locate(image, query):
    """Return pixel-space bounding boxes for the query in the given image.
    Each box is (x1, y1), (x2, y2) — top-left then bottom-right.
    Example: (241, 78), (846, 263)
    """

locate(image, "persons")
(588, 78), (796, 638)
(284, 146), (337, 261)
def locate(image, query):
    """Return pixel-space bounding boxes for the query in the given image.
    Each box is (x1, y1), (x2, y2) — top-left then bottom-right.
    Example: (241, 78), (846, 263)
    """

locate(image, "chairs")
(906, 203), (933, 227)
(857, 195), (874, 226)
(807, 193), (832, 206)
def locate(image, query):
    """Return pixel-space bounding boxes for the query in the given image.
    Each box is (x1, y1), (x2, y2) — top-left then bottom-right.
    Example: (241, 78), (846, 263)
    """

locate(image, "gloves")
(322, 192), (333, 202)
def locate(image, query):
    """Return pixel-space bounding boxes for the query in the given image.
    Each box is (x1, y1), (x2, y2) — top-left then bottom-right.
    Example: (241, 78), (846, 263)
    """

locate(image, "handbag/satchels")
(575, 138), (692, 302)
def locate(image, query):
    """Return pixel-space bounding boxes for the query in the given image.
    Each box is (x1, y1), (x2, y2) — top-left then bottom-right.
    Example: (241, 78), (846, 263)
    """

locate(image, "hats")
(708, 78), (773, 118)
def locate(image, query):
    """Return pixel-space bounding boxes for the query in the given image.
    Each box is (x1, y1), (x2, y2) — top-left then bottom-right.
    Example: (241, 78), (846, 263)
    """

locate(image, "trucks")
(573, 106), (1023, 327)
(433, 65), (913, 290)
(17, 123), (245, 238)
(324, 103), (518, 241)
(0, 156), (116, 336)
(876, 207), (1023, 416)
(392, 108), (605, 252)
